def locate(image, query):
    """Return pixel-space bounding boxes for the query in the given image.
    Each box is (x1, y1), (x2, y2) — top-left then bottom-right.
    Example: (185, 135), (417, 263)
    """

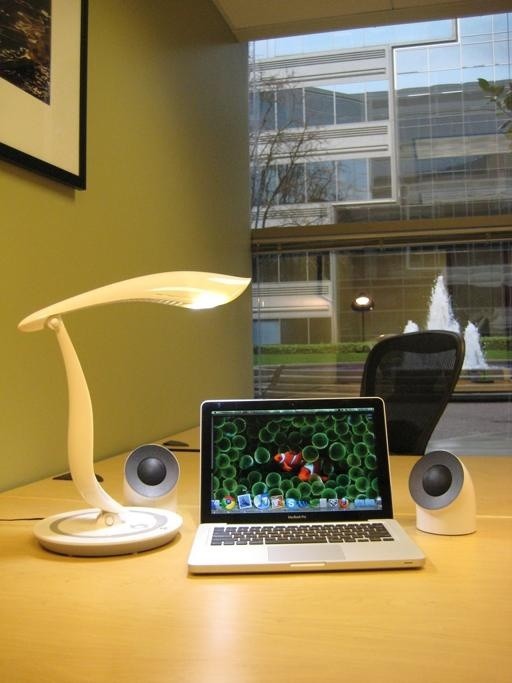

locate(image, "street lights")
(351, 290), (374, 342)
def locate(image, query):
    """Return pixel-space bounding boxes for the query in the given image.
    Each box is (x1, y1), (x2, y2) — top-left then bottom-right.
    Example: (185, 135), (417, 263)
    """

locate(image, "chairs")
(359, 329), (468, 455)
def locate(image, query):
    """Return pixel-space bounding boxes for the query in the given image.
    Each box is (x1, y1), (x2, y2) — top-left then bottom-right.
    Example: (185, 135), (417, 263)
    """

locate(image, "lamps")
(16, 270), (250, 554)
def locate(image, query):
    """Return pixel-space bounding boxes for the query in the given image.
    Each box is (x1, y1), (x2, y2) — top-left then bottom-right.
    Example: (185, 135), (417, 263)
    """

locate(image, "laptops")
(187, 397), (426, 574)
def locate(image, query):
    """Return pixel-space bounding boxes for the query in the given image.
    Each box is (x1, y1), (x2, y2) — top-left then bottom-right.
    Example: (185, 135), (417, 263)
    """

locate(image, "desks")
(2, 417), (512, 682)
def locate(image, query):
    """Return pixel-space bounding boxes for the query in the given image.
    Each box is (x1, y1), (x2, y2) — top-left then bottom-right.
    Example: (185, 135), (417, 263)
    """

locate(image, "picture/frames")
(0, 0), (92, 189)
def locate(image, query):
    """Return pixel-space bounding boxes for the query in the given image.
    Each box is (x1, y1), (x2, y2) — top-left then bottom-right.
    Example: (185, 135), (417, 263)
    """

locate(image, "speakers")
(123, 444), (180, 513)
(408, 450), (476, 536)
